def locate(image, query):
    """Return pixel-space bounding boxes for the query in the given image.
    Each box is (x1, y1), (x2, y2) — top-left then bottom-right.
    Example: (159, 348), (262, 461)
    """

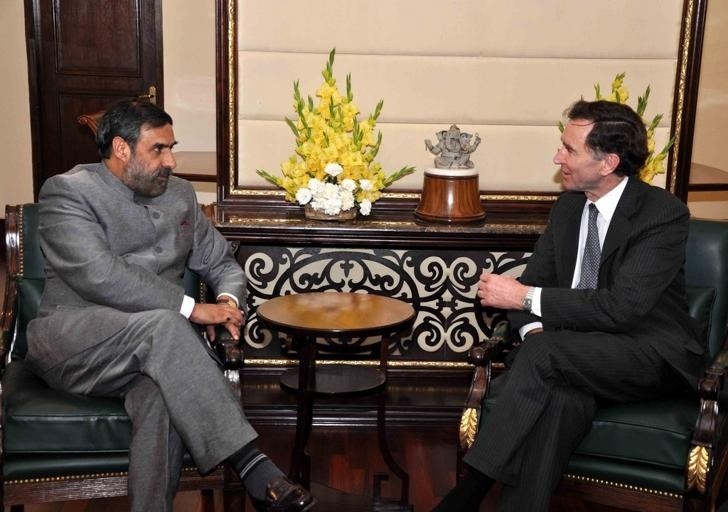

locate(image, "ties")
(575, 204), (601, 289)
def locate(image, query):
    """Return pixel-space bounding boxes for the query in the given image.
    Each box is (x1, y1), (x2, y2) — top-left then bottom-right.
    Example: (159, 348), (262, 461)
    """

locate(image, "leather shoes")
(246, 477), (317, 512)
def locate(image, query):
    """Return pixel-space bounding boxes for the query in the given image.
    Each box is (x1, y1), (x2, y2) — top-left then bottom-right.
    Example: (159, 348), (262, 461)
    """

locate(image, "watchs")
(521, 285), (536, 312)
(218, 296), (238, 308)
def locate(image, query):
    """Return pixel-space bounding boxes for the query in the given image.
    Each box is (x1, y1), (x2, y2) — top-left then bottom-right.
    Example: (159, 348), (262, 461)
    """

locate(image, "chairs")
(0, 200), (249, 511)
(447, 214), (728, 510)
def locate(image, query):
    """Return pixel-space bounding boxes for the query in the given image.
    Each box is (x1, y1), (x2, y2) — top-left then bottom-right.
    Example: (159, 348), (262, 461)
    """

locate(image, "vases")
(300, 191), (359, 223)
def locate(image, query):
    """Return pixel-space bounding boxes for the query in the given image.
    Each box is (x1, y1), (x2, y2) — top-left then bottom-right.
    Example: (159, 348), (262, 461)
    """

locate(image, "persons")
(426, 95), (713, 512)
(22, 96), (318, 512)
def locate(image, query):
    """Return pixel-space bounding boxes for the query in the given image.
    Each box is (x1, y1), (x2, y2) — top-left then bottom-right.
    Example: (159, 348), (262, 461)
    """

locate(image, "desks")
(212, 202), (567, 440)
(255, 292), (417, 508)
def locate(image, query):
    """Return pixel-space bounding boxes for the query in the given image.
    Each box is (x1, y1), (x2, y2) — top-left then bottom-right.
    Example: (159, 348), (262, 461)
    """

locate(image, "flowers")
(254, 45), (418, 217)
(554, 70), (677, 188)
